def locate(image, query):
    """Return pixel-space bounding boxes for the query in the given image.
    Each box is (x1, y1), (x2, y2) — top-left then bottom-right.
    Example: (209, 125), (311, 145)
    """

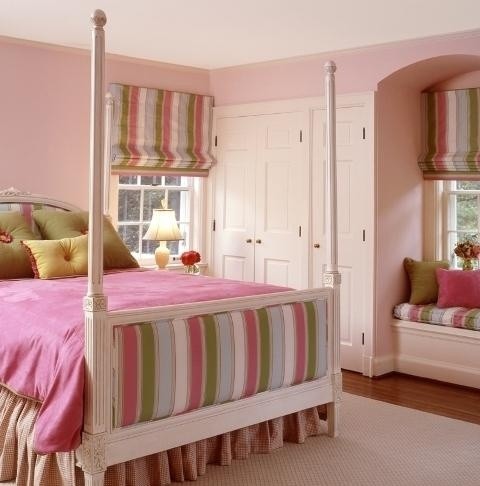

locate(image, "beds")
(0, 7), (342, 485)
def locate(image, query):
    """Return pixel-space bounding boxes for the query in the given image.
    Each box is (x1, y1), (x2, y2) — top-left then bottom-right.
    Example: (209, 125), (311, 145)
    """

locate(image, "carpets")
(177, 388), (479, 485)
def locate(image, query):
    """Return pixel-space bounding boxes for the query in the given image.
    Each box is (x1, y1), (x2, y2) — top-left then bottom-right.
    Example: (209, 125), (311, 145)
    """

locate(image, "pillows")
(402, 256), (480, 310)
(0, 210), (140, 281)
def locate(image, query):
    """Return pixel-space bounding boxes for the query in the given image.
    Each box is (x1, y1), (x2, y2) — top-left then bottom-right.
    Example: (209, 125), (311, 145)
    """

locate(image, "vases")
(184, 263), (200, 275)
(461, 257), (474, 271)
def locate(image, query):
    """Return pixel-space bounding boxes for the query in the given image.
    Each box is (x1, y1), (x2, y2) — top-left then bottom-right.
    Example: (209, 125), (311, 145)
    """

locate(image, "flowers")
(453, 240), (480, 268)
(180, 251), (201, 273)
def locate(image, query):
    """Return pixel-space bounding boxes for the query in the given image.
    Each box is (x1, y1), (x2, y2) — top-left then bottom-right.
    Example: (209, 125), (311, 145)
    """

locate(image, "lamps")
(142, 209), (185, 271)
